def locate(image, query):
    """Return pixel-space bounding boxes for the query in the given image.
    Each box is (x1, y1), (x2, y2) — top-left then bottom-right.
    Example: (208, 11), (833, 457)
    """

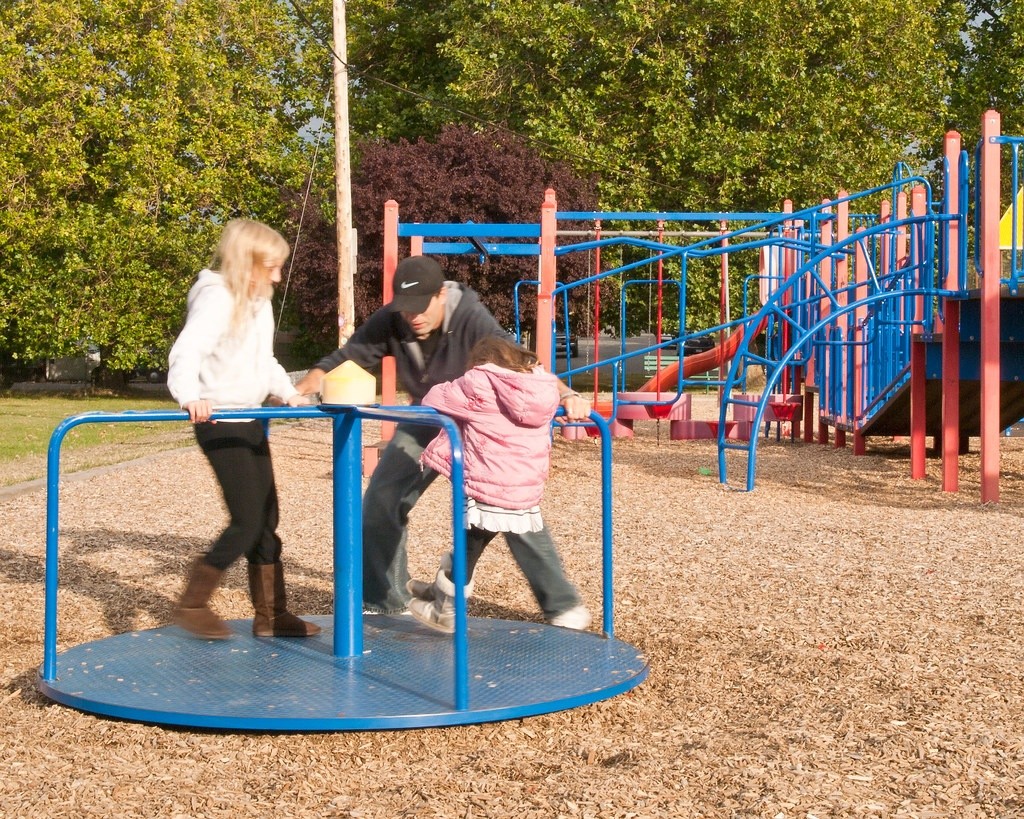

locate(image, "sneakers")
(547, 604), (590, 632)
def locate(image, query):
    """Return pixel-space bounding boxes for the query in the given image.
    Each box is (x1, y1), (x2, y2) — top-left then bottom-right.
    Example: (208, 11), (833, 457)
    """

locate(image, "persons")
(157, 218), (325, 641)
(274, 253), (599, 636)
(403, 334), (562, 635)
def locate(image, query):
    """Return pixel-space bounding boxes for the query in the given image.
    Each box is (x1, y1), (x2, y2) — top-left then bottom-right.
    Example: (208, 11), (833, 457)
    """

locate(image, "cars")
(556, 332), (578, 358)
(656, 332), (713, 360)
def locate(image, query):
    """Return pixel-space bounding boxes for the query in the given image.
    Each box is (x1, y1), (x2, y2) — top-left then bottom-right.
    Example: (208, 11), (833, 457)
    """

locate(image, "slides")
(591, 305), (778, 418)
(857, 332), (1023, 436)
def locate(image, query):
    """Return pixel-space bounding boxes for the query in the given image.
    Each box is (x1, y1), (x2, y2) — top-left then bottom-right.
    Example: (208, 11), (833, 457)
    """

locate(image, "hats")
(388, 256), (444, 313)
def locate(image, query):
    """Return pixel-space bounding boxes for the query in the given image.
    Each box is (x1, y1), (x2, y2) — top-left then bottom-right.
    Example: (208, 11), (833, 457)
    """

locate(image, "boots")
(173, 557), (233, 641)
(406, 570), (474, 634)
(246, 563), (324, 637)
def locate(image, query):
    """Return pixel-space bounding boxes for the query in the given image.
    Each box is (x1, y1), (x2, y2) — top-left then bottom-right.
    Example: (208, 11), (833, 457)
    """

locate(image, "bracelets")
(561, 390), (584, 400)
